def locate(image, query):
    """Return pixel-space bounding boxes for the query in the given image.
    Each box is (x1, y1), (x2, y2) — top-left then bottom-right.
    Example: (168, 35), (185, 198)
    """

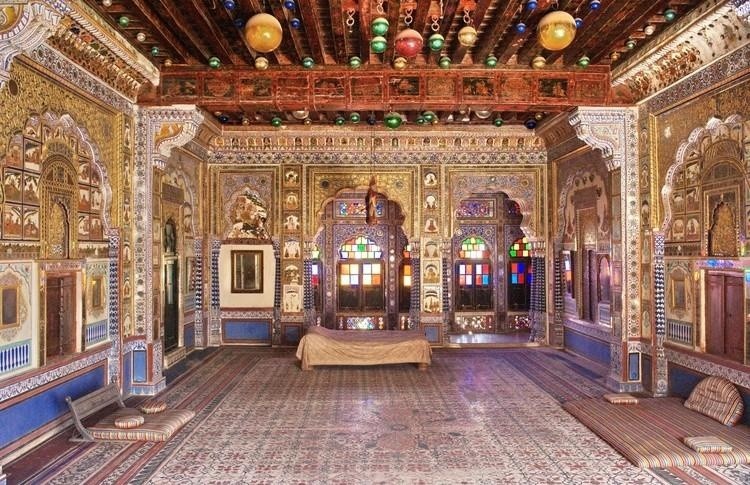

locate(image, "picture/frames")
(558, 248), (575, 299)
(669, 274), (689, 312)
(229, 250), (264, 295)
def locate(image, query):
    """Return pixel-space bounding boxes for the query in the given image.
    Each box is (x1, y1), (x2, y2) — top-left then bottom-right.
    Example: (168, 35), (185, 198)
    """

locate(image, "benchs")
(296, 323), (434, 373)
